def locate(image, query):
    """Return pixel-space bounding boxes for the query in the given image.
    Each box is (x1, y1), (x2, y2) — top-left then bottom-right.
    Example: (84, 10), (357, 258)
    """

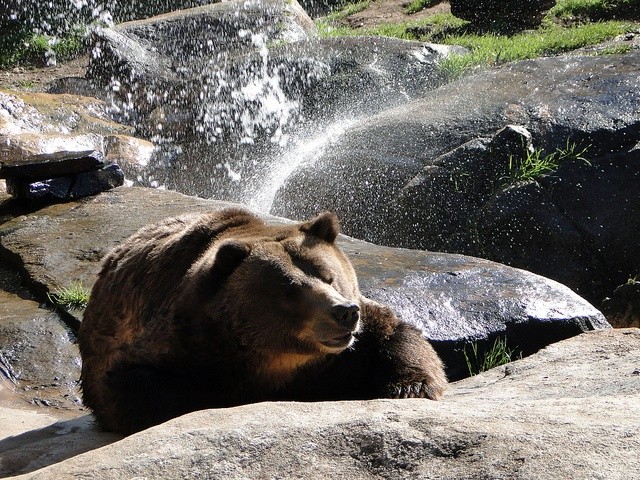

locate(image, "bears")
(73, 207), (449, 434)
